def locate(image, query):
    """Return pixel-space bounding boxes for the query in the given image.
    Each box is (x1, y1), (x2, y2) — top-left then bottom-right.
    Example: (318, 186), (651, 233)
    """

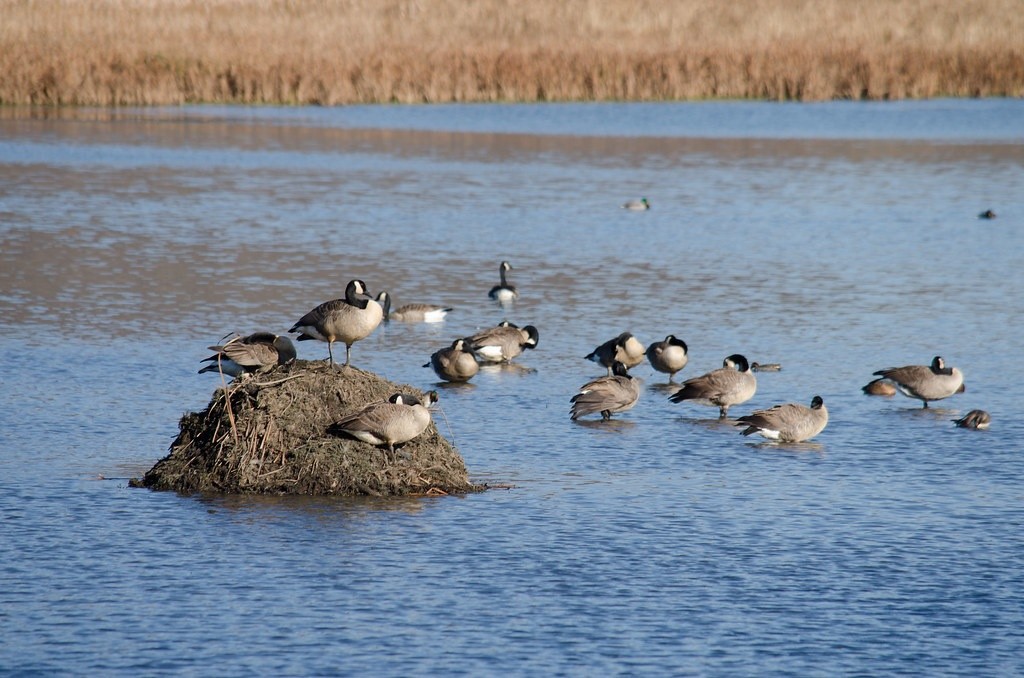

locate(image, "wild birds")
(862, 356), (992, 430)
(198, 332), (297, 378)
(734, 394), (829, 442)
(488, 261), (518, 301)
(325, 393), (432, 464)
(669, 355), (781, 418)
(569, 331), (688, 420)
(620, 198), (650, 210)
(422, 322), (539, 382)
(374, 292), (453, 321)
(288, 280), (384, 371)
